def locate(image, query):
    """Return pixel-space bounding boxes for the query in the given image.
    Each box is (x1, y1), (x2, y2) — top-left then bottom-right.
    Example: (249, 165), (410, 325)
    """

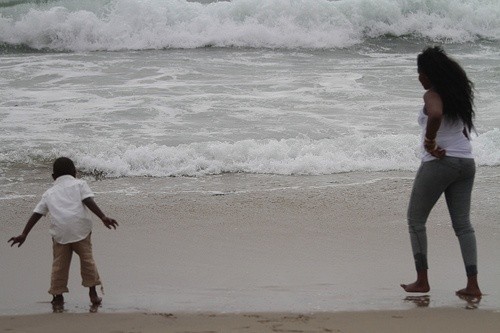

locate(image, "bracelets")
(423, 136), (437, 152)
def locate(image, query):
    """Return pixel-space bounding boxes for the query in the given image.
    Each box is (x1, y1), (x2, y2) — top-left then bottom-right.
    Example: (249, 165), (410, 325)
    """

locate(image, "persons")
(9, 157), (118, 313)
(400, 47), (481, 296)
